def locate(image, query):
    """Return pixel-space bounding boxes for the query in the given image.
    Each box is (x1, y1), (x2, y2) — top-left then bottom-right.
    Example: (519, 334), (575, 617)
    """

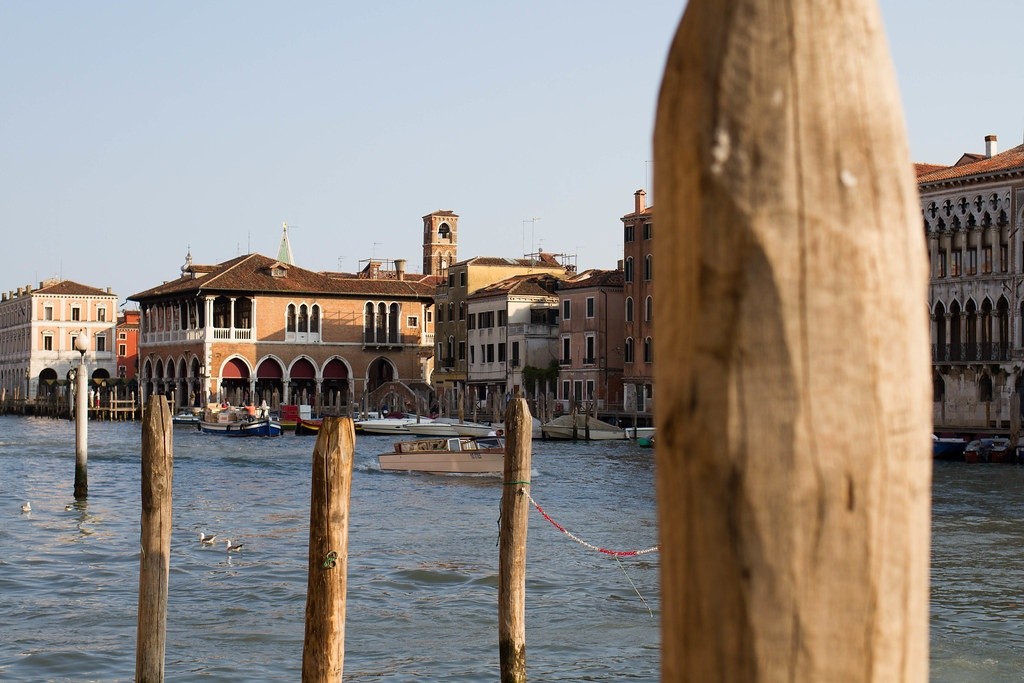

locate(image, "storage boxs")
(281, 406), (298, 420)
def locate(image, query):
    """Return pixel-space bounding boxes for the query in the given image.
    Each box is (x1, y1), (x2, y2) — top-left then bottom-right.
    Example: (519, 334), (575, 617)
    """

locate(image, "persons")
(221, 392), (267, 422)
(95, 391), (101, 408)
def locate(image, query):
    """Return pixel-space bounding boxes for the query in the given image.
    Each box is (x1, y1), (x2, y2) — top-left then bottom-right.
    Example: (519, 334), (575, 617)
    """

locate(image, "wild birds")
(65, 505), (72, 510)
(199, 532), (217, 545)
(225, 539), (244, 553)
(21, 502), (31, 512)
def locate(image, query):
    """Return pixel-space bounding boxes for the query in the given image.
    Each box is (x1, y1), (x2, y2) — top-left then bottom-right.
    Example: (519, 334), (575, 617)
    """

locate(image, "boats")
(541, 412), (626, 440)
(171, 408), (541, 439)
(378, 436), (537, 473)
(934, 434), (1024, 464)
(625, 427), (655, 447)
